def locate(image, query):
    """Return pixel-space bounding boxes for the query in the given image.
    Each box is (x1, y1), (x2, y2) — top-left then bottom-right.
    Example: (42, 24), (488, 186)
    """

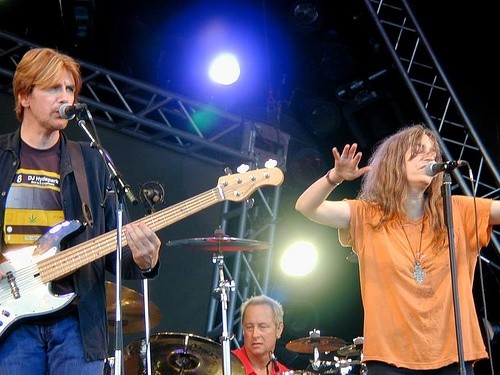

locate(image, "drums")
(308, 359), (361, 375)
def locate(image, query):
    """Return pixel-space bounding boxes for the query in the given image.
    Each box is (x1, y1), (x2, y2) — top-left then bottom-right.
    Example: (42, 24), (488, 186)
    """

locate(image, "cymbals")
(336, 343), (363, 356)
(123, 331), (246, 375)
(286, 336), (346, 353)
(165, 235), (272, 252)
(105, 280), (161, 333)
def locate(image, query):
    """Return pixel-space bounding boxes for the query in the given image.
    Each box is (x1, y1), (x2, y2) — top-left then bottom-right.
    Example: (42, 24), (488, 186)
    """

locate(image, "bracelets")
(326, 170), (338, 186)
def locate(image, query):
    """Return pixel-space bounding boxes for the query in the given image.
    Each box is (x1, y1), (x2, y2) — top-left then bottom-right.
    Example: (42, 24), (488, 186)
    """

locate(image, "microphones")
(270, 352), (279, 371)
(424, 159), (466, 176)
(58, 103), (86, 120)
(138, 180), (167, 210)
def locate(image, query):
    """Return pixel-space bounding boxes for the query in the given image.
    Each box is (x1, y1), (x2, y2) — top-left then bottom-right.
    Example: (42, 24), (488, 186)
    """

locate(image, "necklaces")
(397, 211), (426, 285)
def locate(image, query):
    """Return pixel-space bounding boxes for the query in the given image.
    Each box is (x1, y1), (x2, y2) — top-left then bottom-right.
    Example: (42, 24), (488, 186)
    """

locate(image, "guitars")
(0, 158), (284, 340)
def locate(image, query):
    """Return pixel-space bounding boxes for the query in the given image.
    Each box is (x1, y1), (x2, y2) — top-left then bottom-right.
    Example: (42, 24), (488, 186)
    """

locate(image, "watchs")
(138, 269), (151, 273)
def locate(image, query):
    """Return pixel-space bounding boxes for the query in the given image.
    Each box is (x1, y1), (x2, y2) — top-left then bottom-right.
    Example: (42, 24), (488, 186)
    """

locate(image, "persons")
(230, 296), (292, 375)
(0, 47), (161, 375)
(295, 123), (500, 375)
